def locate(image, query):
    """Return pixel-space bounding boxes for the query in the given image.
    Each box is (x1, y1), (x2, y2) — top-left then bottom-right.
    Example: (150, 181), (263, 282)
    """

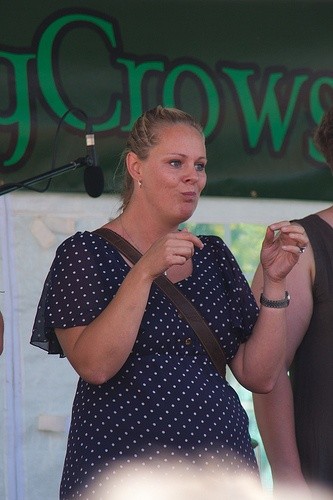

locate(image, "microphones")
(81, 118), (105, 198)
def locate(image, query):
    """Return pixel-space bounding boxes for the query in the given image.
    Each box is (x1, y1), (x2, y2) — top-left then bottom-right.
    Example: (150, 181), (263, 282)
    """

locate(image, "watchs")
(259, 292), (292, 309)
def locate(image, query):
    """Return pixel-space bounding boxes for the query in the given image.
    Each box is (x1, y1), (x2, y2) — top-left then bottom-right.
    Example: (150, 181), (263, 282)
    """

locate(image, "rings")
(296, 244), (306, 254)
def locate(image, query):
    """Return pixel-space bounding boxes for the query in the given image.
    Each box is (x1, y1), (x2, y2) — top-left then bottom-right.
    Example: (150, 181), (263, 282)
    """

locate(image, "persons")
(31, 103), (309, 500)
(249, 105), (333, 499)
